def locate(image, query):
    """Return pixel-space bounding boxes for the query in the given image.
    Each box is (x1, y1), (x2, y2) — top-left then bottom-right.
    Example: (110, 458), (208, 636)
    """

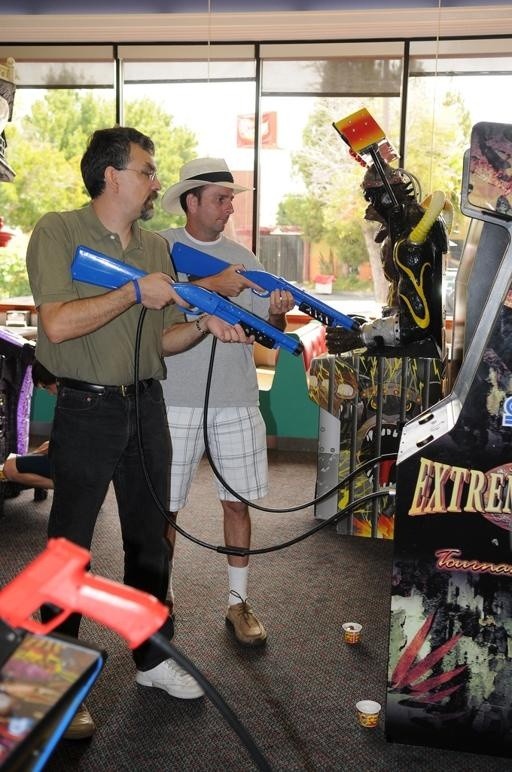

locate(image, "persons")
(25, 127), (256, 742)
(324, 161), (448, 361)
(148, 157), (294, 645)
(0, 360), (63, 489)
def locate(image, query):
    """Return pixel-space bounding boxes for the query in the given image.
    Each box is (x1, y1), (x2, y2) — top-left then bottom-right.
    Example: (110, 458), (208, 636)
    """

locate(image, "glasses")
(114, 167), (158, 183)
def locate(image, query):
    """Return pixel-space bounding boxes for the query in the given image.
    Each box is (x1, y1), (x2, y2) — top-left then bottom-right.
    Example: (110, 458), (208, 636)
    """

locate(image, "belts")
(62, 377), (156, 395)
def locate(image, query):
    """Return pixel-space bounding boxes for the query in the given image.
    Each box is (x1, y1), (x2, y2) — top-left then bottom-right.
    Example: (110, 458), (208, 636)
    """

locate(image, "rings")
(275, 304), (281, 309)
(288, 305), (293, 310)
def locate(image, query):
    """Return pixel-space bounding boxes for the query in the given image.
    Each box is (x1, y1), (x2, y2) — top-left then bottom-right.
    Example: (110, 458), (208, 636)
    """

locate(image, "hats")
(160, 155), (252, 217)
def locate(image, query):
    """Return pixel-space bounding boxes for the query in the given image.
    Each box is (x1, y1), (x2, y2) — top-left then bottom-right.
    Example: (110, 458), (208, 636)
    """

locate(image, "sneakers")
(62, 702), (97, 741)
(133, 659), (206, 700)
(224, 590), (268, 650)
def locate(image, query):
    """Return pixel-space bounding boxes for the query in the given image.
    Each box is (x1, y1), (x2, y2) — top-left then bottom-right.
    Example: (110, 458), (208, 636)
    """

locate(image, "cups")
(355, 700), (381, 728)
(341, 622), (362, 645)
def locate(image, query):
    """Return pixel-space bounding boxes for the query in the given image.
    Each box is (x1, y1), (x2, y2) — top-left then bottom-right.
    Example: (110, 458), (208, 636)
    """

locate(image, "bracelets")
(132, 278), (141, 306)
(197, 312), (209, 337)
